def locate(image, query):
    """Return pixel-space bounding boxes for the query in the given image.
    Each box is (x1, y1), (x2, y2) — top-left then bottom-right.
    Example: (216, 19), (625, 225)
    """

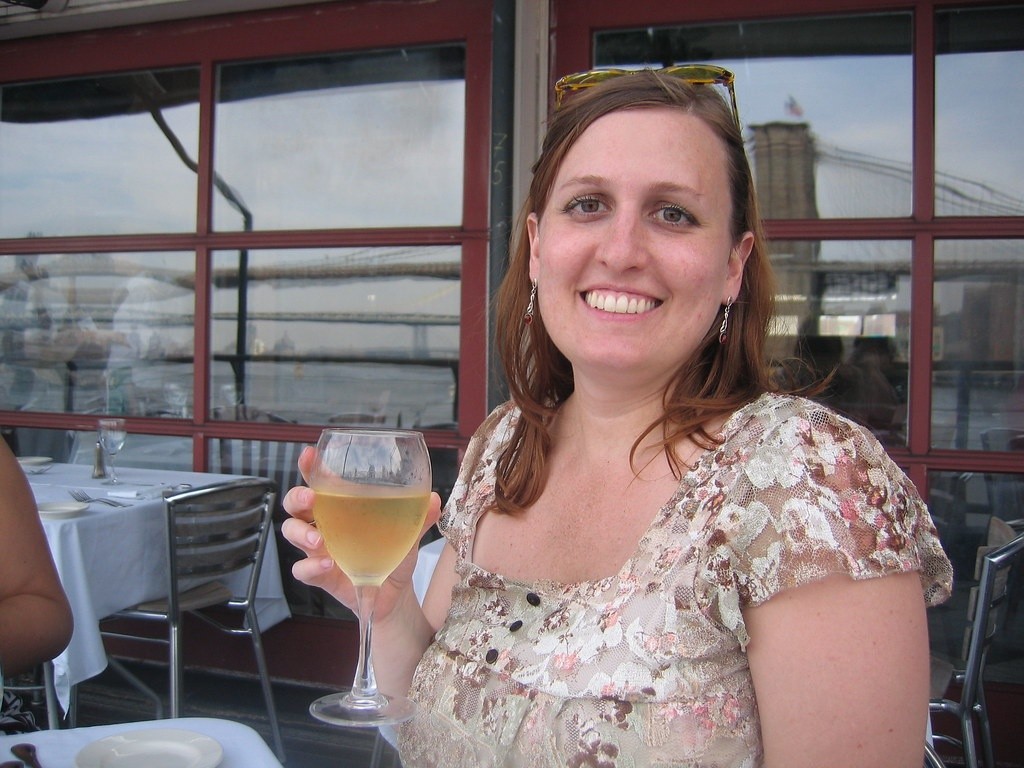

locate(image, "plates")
(74, 729), (223, 768)
(36, 500), (90, 519)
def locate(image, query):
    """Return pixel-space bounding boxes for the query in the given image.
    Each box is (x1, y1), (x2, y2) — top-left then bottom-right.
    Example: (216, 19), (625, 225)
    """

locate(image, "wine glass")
(97, 418), (127, 486)
(308, 429), (432, 727)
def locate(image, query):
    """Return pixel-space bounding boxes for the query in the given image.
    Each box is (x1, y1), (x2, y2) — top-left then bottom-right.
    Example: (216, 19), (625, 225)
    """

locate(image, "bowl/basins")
(15, 456), (53, 475)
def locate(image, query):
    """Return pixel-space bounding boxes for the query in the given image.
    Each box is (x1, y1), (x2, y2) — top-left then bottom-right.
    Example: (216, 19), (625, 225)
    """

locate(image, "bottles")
(92, 441), (105, 479)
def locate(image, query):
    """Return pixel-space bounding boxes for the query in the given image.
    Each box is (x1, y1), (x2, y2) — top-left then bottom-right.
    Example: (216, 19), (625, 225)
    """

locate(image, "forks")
(67, 489), (134, 508)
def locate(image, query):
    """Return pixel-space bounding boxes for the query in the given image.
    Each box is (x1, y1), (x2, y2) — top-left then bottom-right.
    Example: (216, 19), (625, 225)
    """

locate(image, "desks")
(16, 460), (291, 730)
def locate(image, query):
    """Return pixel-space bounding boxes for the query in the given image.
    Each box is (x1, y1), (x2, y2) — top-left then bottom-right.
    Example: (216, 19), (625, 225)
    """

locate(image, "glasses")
(554, 64), (742, 139)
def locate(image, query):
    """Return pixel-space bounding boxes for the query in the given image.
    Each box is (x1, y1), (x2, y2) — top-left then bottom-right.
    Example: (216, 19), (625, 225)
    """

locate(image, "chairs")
(96, 476), (287, 765)
(930, 515), (1024, 768)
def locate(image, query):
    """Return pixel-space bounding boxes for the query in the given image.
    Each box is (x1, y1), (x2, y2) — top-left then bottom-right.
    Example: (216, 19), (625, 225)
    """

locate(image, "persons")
(0, 253), (187, 417)
(281, 66), (954, 768)
(0, 437), (75, 678)
